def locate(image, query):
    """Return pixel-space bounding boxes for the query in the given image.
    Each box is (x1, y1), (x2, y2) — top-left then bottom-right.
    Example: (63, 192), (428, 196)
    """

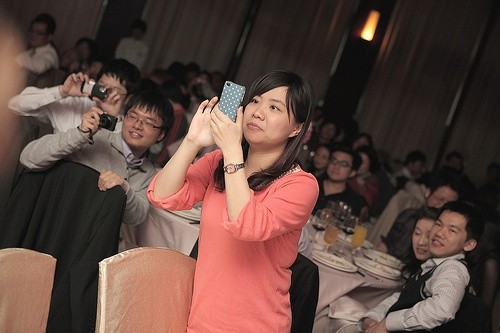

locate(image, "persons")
(146, 71), (319, 333)
(7, 12), (224, 254)
(299, 106), (500, 333)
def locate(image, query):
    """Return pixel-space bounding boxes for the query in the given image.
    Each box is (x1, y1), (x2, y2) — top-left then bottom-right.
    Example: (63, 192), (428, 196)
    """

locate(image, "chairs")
(0, 162), (127, 333)
(385, 252), (500, 333)
(95, 246), (196, 333)
(189, 237), (319, 333)
(0, 247), (57, 333)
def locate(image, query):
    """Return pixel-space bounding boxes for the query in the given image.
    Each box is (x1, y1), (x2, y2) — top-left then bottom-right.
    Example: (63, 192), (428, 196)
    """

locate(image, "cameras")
(92, 113), (118, 131)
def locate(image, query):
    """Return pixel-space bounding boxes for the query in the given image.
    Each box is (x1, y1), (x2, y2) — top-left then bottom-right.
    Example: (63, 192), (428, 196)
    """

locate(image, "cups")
(325, 200), (352, 232)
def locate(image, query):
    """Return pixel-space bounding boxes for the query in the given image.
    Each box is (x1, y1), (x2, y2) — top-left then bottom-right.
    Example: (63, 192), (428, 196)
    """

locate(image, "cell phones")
(80, 79), (109, 100)
(218, 81), (246, 123)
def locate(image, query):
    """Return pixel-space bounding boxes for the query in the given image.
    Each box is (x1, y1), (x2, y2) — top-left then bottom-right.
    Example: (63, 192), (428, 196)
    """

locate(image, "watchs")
(223, 162), (245, 174)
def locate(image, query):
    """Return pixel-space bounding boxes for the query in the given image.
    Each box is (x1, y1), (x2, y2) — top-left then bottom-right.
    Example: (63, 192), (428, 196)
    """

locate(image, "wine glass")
(344, 221), (370, 264)
(322, 222), (340, 252)
(335, 215), (359, 255)
(310, 210), (331, 245)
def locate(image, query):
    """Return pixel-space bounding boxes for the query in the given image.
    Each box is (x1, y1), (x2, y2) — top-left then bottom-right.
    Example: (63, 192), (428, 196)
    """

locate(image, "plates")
(305, 216), (405, 281)
(166, 206), (201, 221)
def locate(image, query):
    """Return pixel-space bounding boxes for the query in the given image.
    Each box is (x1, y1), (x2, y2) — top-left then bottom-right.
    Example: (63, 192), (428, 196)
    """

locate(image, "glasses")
(330, 158), (352, 170)
(29, 28), (47, 36)
(429, 192), (452, 203)
(125, 112), (162, 129)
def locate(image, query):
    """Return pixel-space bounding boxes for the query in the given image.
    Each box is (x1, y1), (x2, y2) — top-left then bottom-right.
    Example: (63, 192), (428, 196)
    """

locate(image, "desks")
(117, 201), (406, 333)
